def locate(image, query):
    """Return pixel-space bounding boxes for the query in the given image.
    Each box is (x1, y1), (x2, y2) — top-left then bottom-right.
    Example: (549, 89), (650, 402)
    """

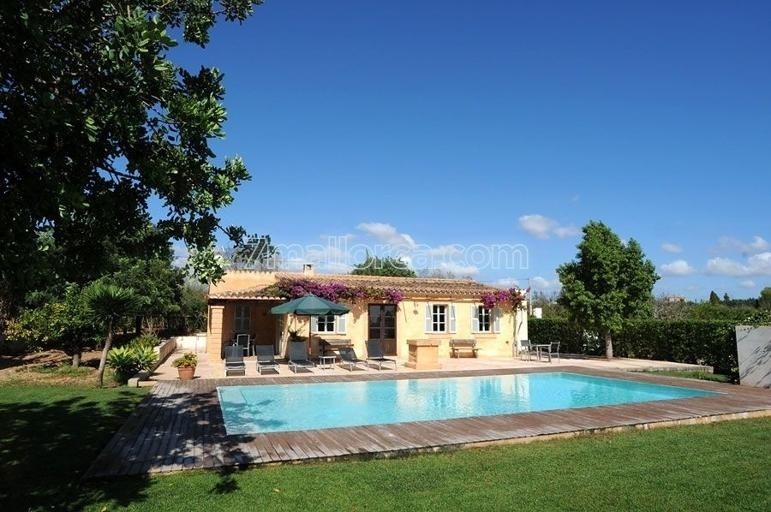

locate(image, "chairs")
(223, 332), (395, 375)
(518, 340), (561, 362)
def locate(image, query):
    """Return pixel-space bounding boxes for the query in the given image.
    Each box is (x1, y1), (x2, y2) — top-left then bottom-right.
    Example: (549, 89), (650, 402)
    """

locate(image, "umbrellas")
(270, 288), (350, 354)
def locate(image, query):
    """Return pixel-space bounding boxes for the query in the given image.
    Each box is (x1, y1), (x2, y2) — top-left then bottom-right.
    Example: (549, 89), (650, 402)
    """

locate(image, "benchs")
(450, 339), (479, 359)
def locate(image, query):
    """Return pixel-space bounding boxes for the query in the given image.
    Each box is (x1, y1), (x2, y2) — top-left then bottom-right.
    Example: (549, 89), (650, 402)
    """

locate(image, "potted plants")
(174, 351), (200, 380)
